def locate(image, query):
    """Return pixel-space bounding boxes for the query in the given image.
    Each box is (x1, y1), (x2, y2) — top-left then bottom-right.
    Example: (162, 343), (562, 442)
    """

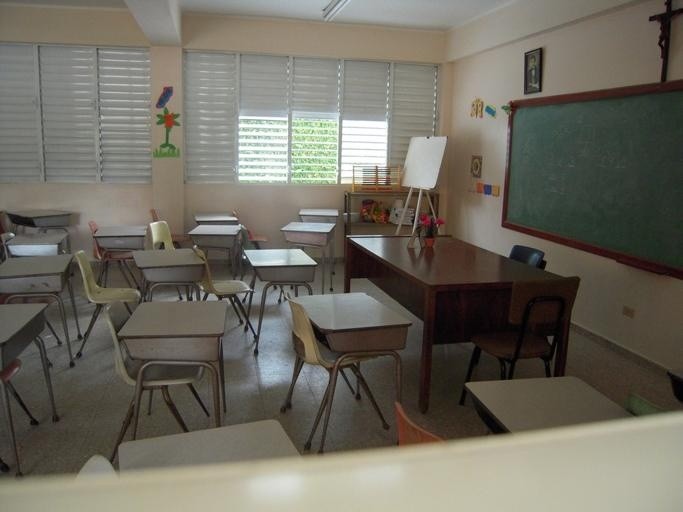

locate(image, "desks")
(343, 234), (580, 412)
(464, 375), (636, 434)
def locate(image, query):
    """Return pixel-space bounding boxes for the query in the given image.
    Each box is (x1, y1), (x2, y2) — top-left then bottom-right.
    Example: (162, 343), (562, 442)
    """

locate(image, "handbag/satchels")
(361, 199), (387, 224)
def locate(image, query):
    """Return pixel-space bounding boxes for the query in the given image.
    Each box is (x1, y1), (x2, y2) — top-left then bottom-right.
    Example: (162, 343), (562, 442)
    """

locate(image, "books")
(388, 207), (415, 226)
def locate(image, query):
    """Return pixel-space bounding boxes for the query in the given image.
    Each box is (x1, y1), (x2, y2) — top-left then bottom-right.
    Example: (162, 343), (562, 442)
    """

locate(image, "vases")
(424, 236), (435, 247)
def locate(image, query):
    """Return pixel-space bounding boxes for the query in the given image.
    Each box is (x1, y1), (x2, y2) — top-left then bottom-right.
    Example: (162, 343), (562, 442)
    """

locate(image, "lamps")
(323, 0), (349, 23)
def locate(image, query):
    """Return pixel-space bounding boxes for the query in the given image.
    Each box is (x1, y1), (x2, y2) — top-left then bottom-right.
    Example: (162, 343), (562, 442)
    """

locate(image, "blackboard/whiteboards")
(501, 79), (683, 279)
(400, 136), (448, 191)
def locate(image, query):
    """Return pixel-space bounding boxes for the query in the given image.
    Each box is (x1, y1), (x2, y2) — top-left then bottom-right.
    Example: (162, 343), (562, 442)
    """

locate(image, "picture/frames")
(523, 47), (542, 94)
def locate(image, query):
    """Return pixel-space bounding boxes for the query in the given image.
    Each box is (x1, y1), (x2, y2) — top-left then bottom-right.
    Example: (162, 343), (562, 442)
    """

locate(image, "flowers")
(155, 107), (179, 145)
(418, 212), (445, 237)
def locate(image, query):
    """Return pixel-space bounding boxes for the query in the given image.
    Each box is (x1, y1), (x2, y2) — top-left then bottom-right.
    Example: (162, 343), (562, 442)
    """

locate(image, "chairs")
(459, 277), (580, 406)
(508, 244), (547, 270)
(393, 399), (443, 445)
(624, 391), (665, 417)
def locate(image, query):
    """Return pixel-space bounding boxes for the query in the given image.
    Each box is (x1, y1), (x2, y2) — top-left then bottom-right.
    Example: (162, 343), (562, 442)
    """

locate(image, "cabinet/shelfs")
(344, 189), (440, 245)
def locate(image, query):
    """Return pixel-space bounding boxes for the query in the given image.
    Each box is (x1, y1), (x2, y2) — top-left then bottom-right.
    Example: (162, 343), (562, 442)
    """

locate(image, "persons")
(526, 55), (539, 89)
